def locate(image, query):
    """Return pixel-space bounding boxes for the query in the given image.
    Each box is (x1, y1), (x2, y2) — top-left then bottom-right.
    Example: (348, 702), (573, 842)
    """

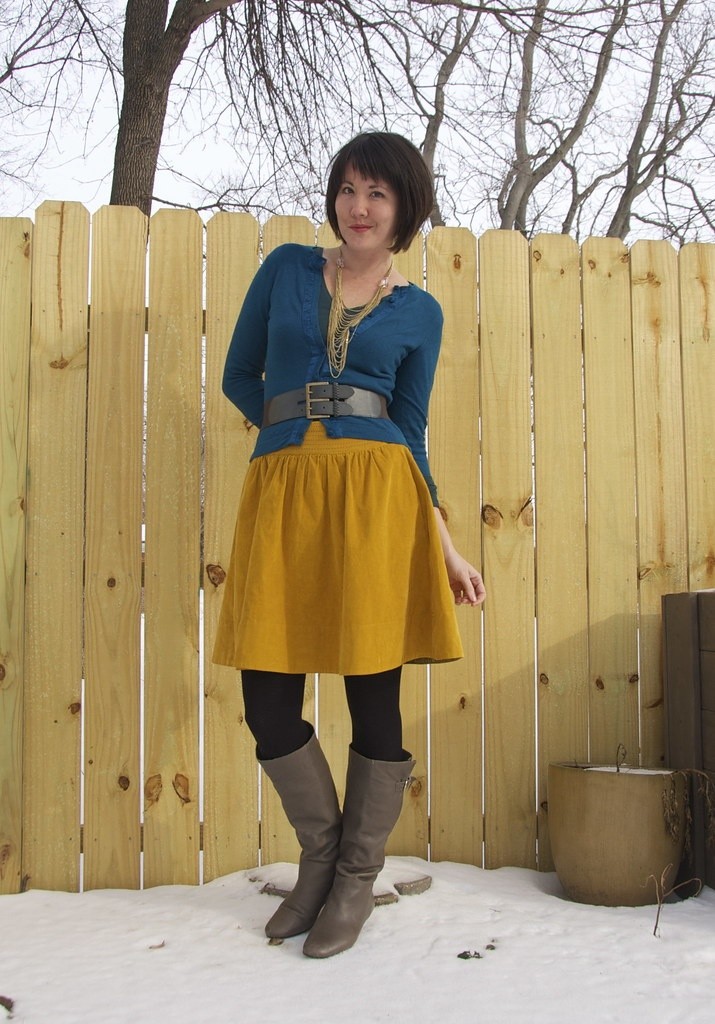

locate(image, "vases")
(543, 757), (692, 910)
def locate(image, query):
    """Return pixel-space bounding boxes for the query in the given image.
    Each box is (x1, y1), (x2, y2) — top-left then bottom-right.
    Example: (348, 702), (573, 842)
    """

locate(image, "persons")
(212, 130), (486, 959)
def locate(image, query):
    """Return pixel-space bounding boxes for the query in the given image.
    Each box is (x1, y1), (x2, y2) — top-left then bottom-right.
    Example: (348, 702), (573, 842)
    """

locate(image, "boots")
(302, 743), (416, 959)
(255, 719), (343, 939)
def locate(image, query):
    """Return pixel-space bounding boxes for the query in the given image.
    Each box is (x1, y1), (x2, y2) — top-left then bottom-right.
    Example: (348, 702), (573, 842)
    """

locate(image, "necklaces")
(326, 246), (394, 379)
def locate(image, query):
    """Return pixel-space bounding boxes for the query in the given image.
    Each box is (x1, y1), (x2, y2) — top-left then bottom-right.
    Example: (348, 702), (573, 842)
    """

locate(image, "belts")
(260, 382), (391, 430)
